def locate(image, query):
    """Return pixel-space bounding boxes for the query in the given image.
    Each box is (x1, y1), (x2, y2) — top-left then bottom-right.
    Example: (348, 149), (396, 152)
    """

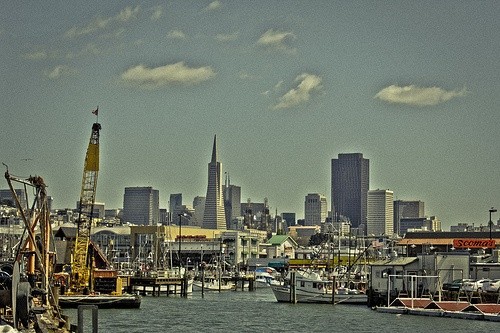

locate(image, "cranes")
(61, 104), (102, 296)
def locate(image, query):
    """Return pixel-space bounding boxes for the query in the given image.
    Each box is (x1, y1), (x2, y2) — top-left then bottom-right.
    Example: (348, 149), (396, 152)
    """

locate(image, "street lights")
(489, 207), (498, 254)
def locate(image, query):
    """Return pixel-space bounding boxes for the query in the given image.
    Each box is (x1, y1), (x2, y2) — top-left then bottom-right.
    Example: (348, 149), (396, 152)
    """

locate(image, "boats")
(268, 232), (368, 302)
(195, 261), (284, 290)
(128, 266), (195, 294)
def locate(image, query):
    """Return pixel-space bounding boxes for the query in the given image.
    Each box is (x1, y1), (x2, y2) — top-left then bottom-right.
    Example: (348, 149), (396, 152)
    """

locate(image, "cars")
(442, 278), (500, 293)
